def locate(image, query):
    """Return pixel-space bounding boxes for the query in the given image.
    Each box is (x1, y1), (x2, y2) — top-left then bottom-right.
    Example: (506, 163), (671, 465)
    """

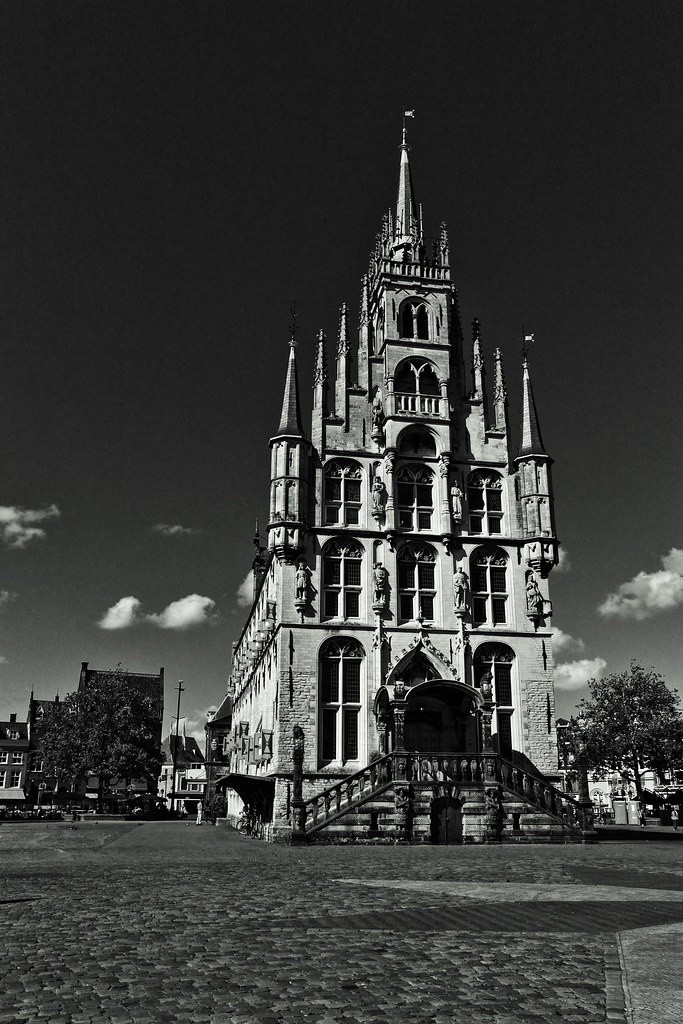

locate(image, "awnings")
(208, 695), (231, 724)
(215, 773), (276, 788)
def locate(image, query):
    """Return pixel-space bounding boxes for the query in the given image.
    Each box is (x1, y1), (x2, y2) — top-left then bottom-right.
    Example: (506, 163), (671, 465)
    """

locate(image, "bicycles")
(237, 807), (260, 839)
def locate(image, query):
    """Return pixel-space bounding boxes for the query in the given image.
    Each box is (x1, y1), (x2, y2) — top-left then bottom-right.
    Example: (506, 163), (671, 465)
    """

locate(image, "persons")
(451, 480), (463, 514)
(453, 567), (467, 609)
(196, 800), (202, 825)
(372, 562), (386, 603)
(525, 575), (540, 610)
(372, 398), (382, 432)
(371, 476), (383, 510)
(671, 807), (678, 831)
(132, 806), (142, 815)
(295, 562), (307, 601)
(640, 806), (646, 828)
(181, 804), (188, 819)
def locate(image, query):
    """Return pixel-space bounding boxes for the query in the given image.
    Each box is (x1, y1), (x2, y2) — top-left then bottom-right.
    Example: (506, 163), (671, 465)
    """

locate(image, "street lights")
(170, 680), (186, 811)
